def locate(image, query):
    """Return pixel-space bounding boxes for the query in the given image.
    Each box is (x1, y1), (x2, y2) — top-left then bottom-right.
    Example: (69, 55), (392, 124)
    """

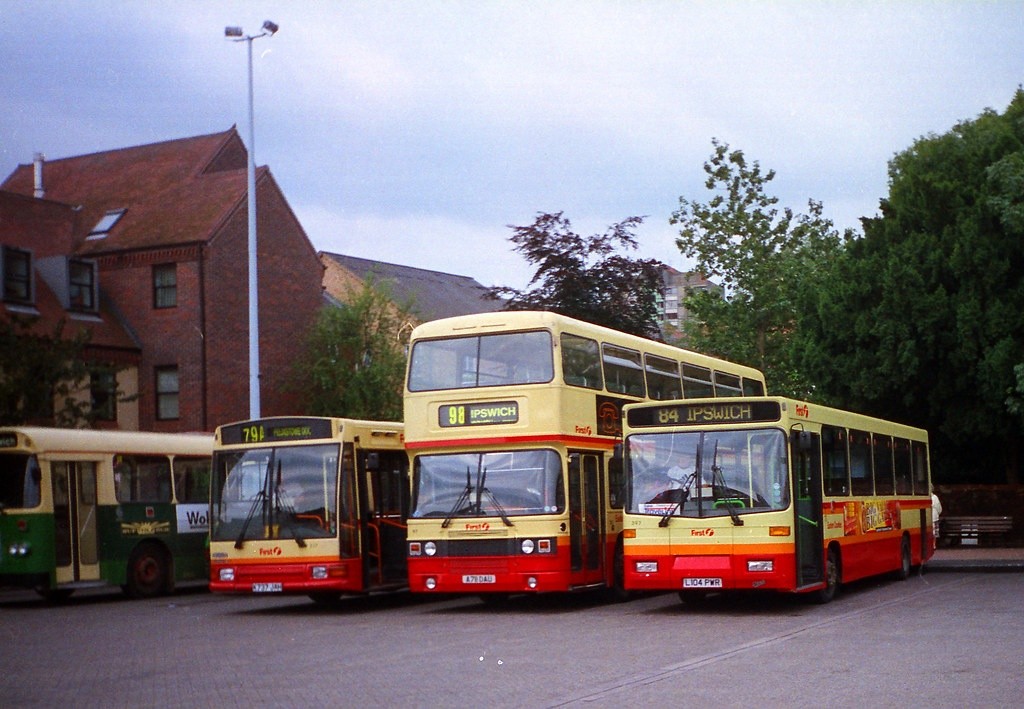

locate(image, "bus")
(611, 396), (935, 612)
(401, 311), (771, 609)
(1, 426), (214, 602)
(208, 414), (405, 608)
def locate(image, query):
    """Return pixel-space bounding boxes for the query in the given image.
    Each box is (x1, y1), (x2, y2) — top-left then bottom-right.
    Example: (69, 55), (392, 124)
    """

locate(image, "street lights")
(223, 20), (280, 424)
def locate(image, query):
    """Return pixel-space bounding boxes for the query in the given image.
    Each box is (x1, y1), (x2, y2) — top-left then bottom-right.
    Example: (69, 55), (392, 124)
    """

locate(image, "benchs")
(942, 516), (1013, 548)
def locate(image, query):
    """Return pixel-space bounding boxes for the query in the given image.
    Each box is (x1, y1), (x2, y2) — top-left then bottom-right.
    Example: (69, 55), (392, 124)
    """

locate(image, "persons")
(271, 469), (305, 511)
(666, 451), (706, 488)
(930, 484), (942, 549)
